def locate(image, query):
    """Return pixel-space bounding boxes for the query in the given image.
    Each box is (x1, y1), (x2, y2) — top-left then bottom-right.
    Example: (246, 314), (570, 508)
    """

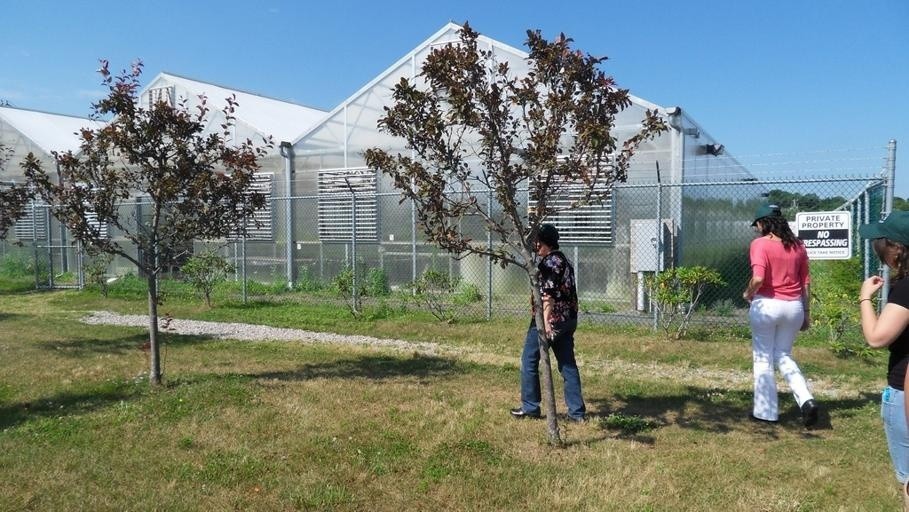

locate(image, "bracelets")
(857, 297), (873, 305)
(804, 308), (810, 313)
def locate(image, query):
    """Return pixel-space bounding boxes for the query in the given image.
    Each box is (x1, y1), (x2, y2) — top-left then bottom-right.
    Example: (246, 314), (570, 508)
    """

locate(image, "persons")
(741, 203), (821, 427)
(857, 210), (909, 492)
(510, 224), (587, 423)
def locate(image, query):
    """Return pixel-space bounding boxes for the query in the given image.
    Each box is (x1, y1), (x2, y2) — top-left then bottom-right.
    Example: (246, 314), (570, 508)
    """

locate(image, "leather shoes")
(564, 413), (585, 425)
(747, 411), (778, 425)
(801, 398), (820, 431)
(510, 406), (541, 419)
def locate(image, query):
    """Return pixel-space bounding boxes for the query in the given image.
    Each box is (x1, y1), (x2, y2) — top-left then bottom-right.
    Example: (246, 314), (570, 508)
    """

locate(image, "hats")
(858, 209), (909, 246)
(750, 203), (780, 227)
(535, 223), (560, 250)
